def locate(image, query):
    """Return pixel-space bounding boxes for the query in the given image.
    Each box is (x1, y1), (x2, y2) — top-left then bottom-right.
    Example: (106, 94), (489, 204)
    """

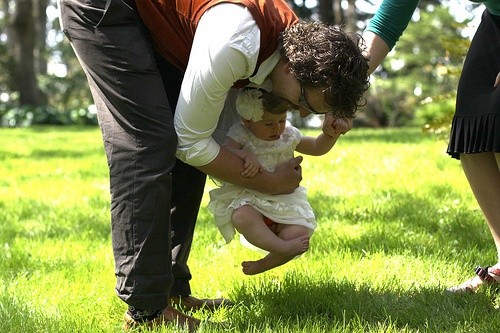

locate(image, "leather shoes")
(124, 304), (211, 333)
(169, 294), (235, 311)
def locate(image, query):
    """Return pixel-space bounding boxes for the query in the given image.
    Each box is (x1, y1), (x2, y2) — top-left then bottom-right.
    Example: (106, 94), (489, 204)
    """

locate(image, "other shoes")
(445, 266), (500, 294)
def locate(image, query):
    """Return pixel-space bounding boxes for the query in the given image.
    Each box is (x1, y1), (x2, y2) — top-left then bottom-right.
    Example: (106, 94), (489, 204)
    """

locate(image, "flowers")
(235, 88), (264, 122)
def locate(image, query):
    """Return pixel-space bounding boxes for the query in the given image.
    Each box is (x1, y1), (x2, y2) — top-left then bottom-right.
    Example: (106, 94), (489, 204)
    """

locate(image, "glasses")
(298, 82), (327, 115)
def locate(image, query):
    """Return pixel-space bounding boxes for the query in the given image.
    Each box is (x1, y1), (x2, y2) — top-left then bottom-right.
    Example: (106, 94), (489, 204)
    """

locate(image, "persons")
(322, 0), (500, 293)
(209, 87), (349, 275)
(57, 0), (369, 333)
